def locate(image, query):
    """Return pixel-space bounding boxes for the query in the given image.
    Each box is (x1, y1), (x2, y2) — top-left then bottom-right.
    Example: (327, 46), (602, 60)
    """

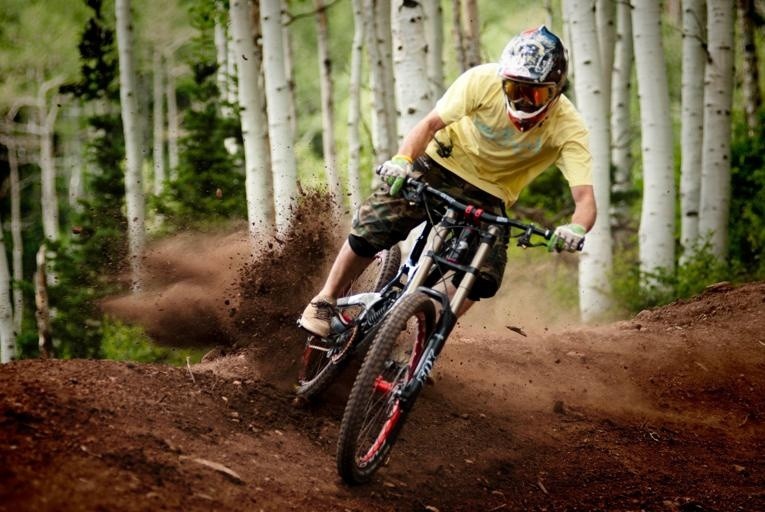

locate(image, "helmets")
(495, 22), (571, 131)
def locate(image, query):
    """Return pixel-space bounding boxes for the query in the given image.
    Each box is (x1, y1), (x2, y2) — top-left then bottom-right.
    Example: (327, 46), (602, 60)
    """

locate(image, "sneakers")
(297, 295), (335, 340)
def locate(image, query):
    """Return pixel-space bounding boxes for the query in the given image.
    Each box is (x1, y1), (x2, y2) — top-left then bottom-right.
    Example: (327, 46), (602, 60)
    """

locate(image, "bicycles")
(291, 163), (590, 483)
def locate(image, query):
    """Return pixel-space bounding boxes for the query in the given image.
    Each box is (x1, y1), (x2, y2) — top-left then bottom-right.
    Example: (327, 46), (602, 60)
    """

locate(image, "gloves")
(543, 221), (590, 256)
(377, 152), (415, 195)
